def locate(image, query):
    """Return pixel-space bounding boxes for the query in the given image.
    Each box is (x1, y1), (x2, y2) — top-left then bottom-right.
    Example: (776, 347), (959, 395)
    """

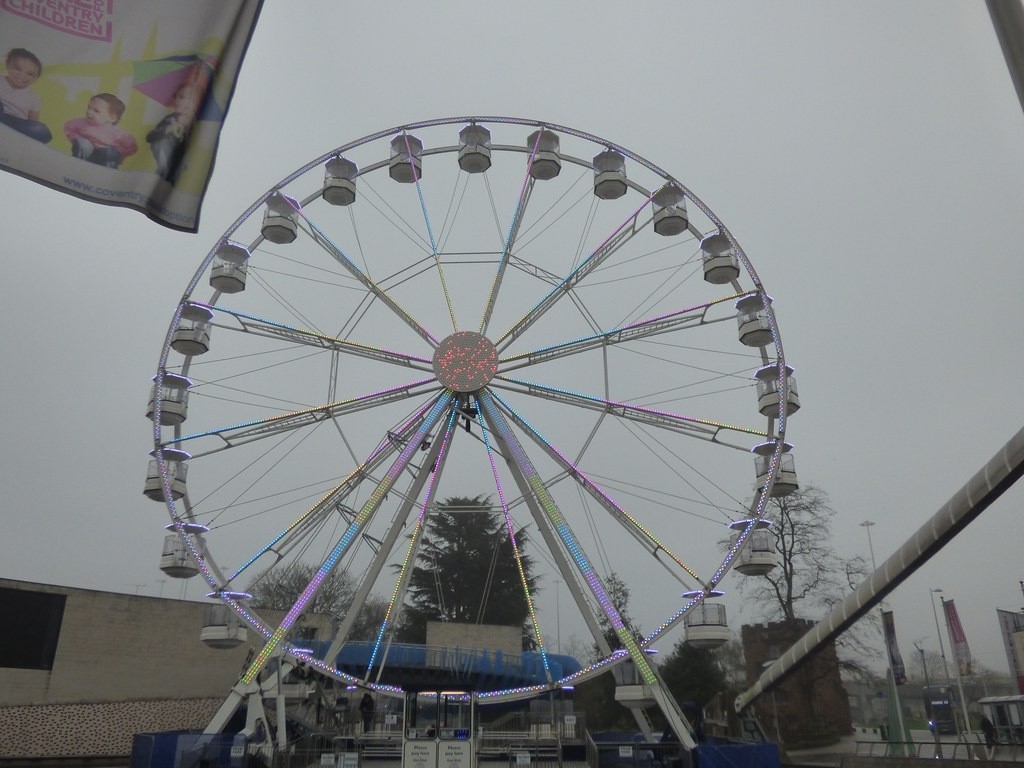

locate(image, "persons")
(0, 48), (53, 144)
(980, 714), (996, 750)
(146, 85), (203, 183)
(65, 93), (137, 169)
(359, 693), (375, 732)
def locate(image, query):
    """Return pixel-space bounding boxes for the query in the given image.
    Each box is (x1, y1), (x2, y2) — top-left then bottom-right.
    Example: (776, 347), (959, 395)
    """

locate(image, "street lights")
(861, 520), (910, 758)
(929, 588), (962, 743)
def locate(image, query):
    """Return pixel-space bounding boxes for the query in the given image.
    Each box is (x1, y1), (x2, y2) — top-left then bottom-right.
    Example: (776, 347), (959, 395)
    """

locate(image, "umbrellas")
(130, 50), (228, 121)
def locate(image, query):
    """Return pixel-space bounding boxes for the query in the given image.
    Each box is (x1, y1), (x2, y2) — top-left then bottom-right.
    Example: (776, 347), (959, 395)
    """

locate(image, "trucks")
(923, 685), (962, 734)
